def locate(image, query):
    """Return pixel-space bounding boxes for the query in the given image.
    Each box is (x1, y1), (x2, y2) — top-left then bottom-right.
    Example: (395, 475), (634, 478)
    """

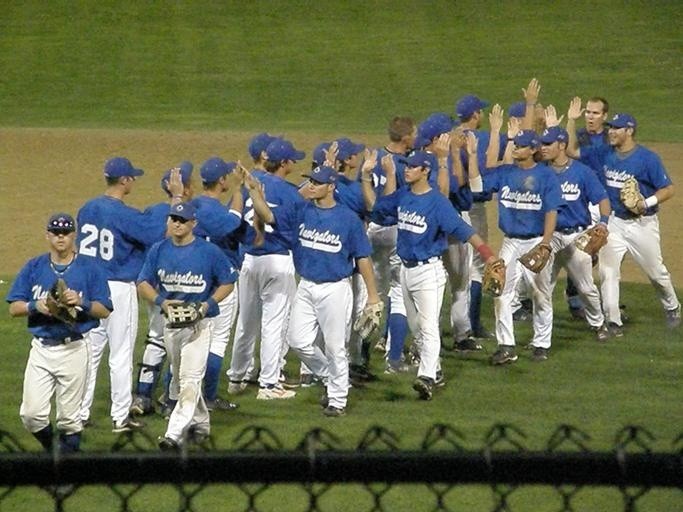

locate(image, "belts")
(34, 335), (83, 346)
(506, 233), (543, 239)
(401, 256), (444, 267)
(615, 211), (656, 221)
(555, 225), (589, 235)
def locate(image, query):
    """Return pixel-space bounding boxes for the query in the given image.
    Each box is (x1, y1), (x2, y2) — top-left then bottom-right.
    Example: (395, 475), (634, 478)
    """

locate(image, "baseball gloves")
(482, 257), (506, 297)
(519, 243), (552, 273)
(46, 278), (83, 327)
(352, 300), (385, 346)
(620, 178), (647, 216)
(167, 298), (202, 329)
(574, 223), (609, 255)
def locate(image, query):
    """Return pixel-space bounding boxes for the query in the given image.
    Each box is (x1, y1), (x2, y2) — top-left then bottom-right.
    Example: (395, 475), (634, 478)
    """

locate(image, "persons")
(6, 78), (681, 452)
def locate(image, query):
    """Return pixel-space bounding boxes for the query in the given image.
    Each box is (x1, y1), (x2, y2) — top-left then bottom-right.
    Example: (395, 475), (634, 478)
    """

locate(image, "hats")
(248, 132), (281, 159)
(47, 213), (77, 231)
(200, 157), (237, 183)
(265, 138), (307, 161)
(302, 166), (338, 185)
(102, 157), (145, 177)
(168, 202), (195, 221)
(312, 143), (333, 163)
(396, 95), (636, 167)
(161, 161), (192, 191)
(333, 138), (364, 160)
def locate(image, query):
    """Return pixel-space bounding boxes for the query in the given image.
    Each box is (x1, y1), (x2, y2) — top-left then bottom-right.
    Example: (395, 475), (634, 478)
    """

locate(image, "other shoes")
(158, 439), (177, 447)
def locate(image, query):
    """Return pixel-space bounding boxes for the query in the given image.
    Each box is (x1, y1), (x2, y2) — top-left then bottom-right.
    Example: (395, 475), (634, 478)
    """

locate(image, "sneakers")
(533, 347), (548, 362)
(453, 336), (485, 352)
(414, 376), (434, 400)
(278, 370), (300, 388)
(511, 307), (533, 321)
(227, 378), (249, 395)
(489, 345), (517, 366)
(346, 335), (422, 388)
(319, 394), (329, 408)
(667, 302), (680, 332)
(467, 327), (495, 339)
(203, 393), (241, 411)
(256, 383), (296, 400)
(111, 417), (144, 432)
(435, 371), (446, 389)
(570, 304), (630, 339)
(439, 335), (446, 358)
(323, 406), (346, 418)
(129, 390), (179, 418)
(300, 374), (322, 387)
